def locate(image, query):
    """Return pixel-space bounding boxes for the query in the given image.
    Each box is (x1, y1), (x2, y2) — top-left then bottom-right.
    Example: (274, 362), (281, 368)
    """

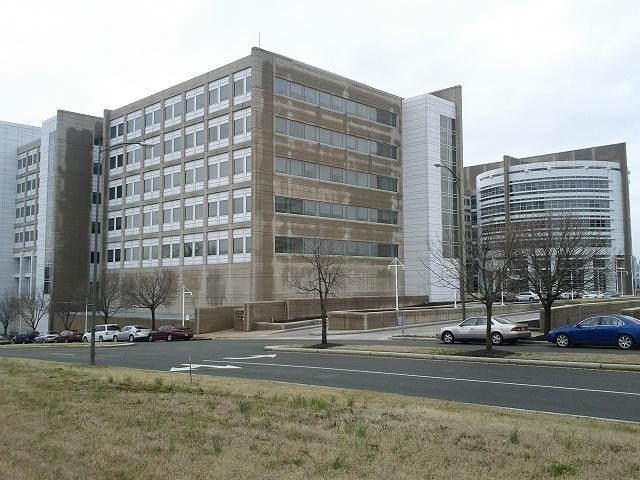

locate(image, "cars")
(435, 315), (532, 346)
(488, 287), (620, 303)
(0, 322), (195, 347)
(546, 313), (640, 351)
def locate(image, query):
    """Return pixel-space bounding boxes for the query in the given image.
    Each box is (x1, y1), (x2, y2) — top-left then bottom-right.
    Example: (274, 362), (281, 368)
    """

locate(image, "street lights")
(495, 260), (512, 306)
(88, 138), (156, 367)
(613, 266), (629, 299)
(433, 162), (467, 321)
(387, 256), (406, 311)
(565, 266), (580, 301)
(444, 257), (462, 309)
(85, 298), (94, 333)
(182, 283), (193, 327)
